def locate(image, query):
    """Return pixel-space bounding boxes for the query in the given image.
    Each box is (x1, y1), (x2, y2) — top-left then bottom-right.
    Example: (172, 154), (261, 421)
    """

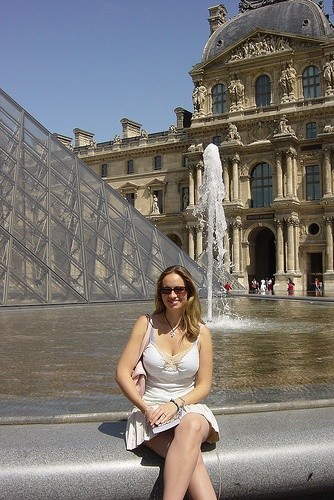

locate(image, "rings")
(162, 413), (168, 417)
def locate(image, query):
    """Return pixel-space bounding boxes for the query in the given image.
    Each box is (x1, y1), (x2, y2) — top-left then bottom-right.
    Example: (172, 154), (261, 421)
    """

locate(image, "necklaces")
(162, 309), (185, 338)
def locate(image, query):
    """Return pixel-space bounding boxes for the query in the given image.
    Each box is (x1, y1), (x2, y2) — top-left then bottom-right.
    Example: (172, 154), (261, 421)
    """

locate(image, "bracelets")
(169, 395), (187, 411)
(143, 404), (154, 417)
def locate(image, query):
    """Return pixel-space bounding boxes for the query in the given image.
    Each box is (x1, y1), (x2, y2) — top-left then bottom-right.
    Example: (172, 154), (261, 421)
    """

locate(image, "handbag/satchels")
(130, 315), (153, 398)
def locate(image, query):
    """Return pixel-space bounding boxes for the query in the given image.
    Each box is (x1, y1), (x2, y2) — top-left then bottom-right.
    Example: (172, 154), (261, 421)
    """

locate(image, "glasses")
(160, 286), (187, 294)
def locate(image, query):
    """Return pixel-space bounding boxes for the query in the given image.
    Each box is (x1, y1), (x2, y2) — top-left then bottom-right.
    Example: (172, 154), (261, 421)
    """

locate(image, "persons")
(115, 264), (224, 500)
(152, 192), (160, 212)
(189, 60), (334, 120)
(68, 122), (181, 155)
(223, 279), (233, 292)
(249, 275), (276, 294)
(229, 33), (290, 62)
(314, 278), (321, 297)
(185, 114), (334, 159)
